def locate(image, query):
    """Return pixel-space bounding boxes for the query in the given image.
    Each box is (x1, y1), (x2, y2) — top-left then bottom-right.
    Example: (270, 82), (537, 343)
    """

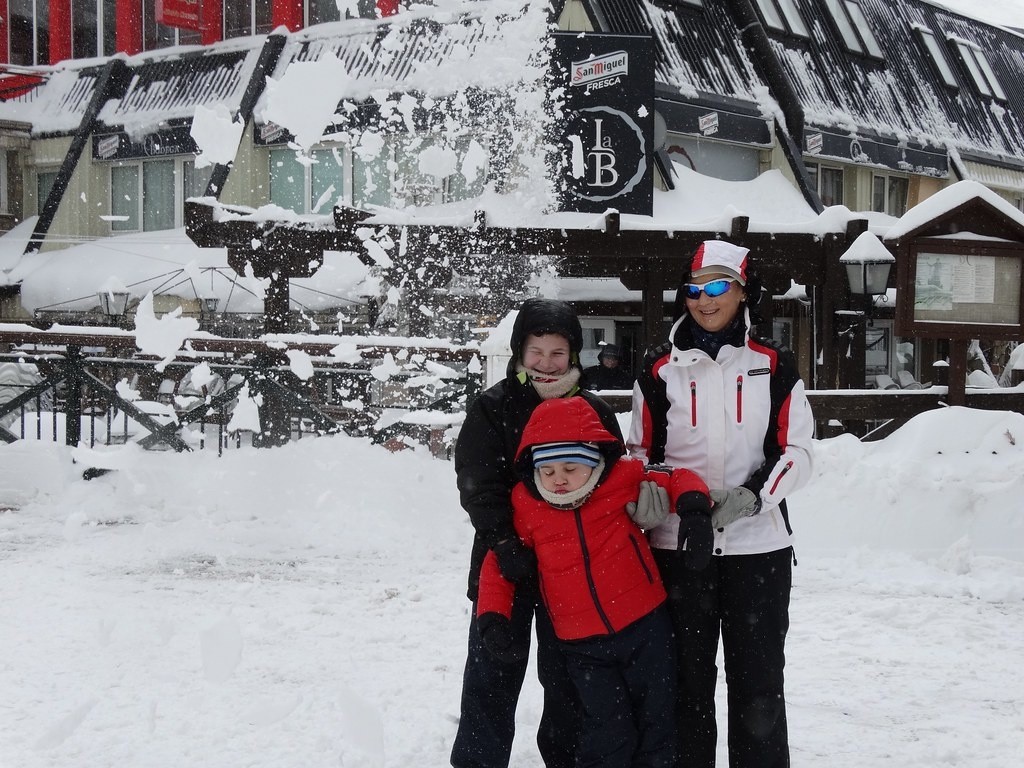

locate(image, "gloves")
(709, 486), (759, 529)
(492, 537), (536, 582)
(676, 491), (715, 571)
(626, 480), (670, 530)
(482, 623), (527, 663)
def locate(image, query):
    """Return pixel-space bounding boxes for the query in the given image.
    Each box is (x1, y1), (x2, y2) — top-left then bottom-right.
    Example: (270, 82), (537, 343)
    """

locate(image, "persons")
(584, 345), (635, 414)
(628, 241), (815, 767)
(450, 298), (630, 768)
(474, 397), (714, 768)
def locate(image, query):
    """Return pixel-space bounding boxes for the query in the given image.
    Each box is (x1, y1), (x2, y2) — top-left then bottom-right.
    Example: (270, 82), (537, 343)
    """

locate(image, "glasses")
(682, 278), (736, 299)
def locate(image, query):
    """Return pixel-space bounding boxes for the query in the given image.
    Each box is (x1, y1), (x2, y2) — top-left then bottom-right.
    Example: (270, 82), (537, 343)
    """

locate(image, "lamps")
(840, 229), (897, 327)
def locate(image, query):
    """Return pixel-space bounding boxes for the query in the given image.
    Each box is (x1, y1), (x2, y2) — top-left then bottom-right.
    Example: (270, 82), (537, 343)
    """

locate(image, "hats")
(598, 343), (622, 362)
(690, 240), (751, 286)
(531, 441), (600, 467)
